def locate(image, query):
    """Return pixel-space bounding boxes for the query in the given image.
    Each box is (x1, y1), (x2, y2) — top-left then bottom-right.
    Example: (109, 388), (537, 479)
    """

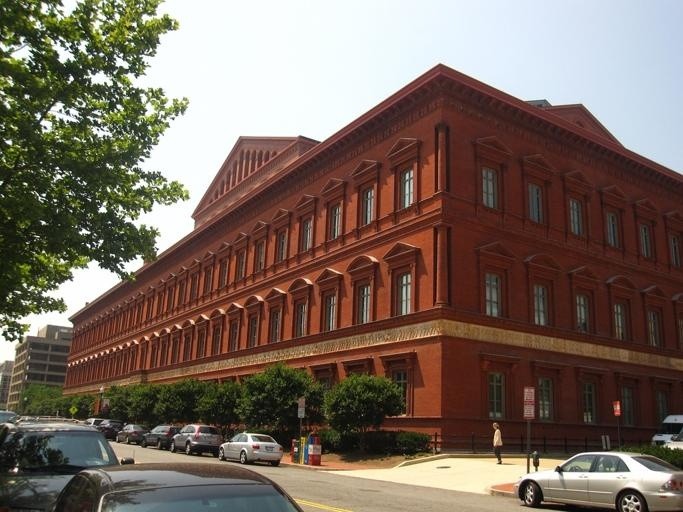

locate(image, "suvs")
(0, 416), (133, 512)
(141, 426), (181, 451)
(170, 425), (222, 458)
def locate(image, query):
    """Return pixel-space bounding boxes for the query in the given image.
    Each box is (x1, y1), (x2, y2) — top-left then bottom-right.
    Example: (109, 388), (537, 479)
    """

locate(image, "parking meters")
(530, 452), (539, 472)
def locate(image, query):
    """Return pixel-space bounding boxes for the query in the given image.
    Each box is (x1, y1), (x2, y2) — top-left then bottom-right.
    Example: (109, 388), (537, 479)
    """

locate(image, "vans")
(651, 414), (682, 446)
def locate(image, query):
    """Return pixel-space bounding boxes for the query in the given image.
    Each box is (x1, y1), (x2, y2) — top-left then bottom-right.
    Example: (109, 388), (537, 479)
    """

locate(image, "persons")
(492, 422), (503, 463)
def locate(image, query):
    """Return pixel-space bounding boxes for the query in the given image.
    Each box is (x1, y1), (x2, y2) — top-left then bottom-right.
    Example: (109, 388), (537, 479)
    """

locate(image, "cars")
(218, 433), (283, 467)
(0, 410), (149, 445)
(513, 450), (682, 512)
(660, 429), (682, 450)
(41, 462), (305, 512)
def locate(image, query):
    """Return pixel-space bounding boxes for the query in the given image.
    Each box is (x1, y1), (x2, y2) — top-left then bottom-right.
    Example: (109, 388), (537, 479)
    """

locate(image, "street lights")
(23, 397), (28, 407)
(97, 385), (104, 415)
